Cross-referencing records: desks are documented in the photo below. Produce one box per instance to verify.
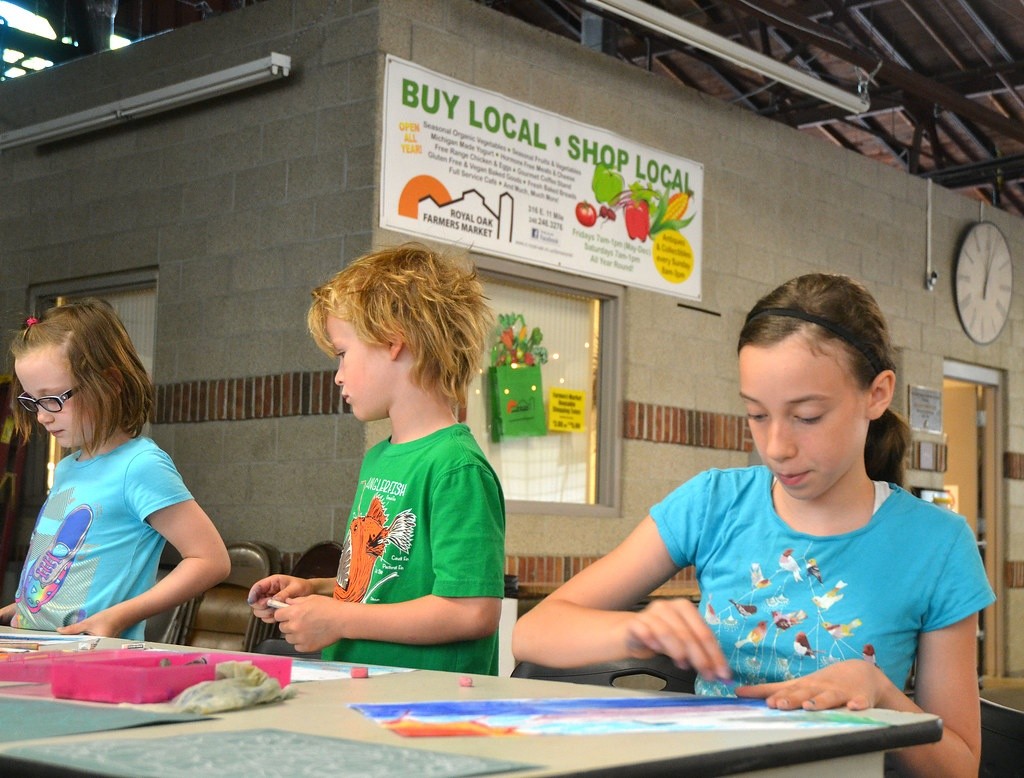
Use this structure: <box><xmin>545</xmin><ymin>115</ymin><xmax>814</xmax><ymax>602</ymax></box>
<box><xmin>0</xmin><ymin>626</ymin><xmax>943</xmax><ymax>778</ymax></box>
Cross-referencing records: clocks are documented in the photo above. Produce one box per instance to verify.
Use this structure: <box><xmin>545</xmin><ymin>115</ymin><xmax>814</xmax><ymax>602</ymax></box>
<box><xmin>955</xmin><ymin>222</ymin><xmax>1012</xmax><ymax>344</ymax></box>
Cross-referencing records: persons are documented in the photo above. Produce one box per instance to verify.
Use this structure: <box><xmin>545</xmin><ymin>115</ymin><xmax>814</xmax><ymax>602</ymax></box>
<box><xmin>512</xmin><ymin>273</ymin><xmax>996</xmax><ymax>778</ymax></box>
<box><xmin>247</xmin><ymin>242</ymin><xmax>508</xmax><ymax>678</ymax></box>
<box><xmin>0</xmin><ymin>297</ymin><xmax>231</xmax><ymax>637</ymax></box>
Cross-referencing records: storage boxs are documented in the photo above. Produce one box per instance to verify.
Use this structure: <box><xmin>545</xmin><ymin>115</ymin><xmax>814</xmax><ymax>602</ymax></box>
<box><xmin>0</xmin><ymin>648</ymin><xmax>293</xmax><ymax>706</ymax></box>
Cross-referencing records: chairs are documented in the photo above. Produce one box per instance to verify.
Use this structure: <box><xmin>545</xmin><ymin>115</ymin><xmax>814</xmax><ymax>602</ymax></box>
<box><xmin>289</xmin><ymin>540</ymin><xmax>345</xmax><ymax>579</ymax></box>
<box><xmin>881</xmin><ymin>696</ymin><xmax>1024</xmax><ymax>778</ymax></box>
<box><xmin>163</xmin><ymin>540</ymin><xmax>282</xmax><ymax>653</ymax></box>
<box><xmin>509</xmin><ymin>650</ymin><xmax>698</xmax><ymax>696</ymax></box>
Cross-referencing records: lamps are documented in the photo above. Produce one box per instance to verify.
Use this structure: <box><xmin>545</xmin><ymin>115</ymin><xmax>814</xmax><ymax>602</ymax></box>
<box><xmin>0</xmin><ymin>52</ymin><xmax>290</xmax><ymax>151</ymax></box>
<box><xmin>586</xmin><ymin>0</ymin><xmax>870</xmax><ymax>114</ymax></box>
